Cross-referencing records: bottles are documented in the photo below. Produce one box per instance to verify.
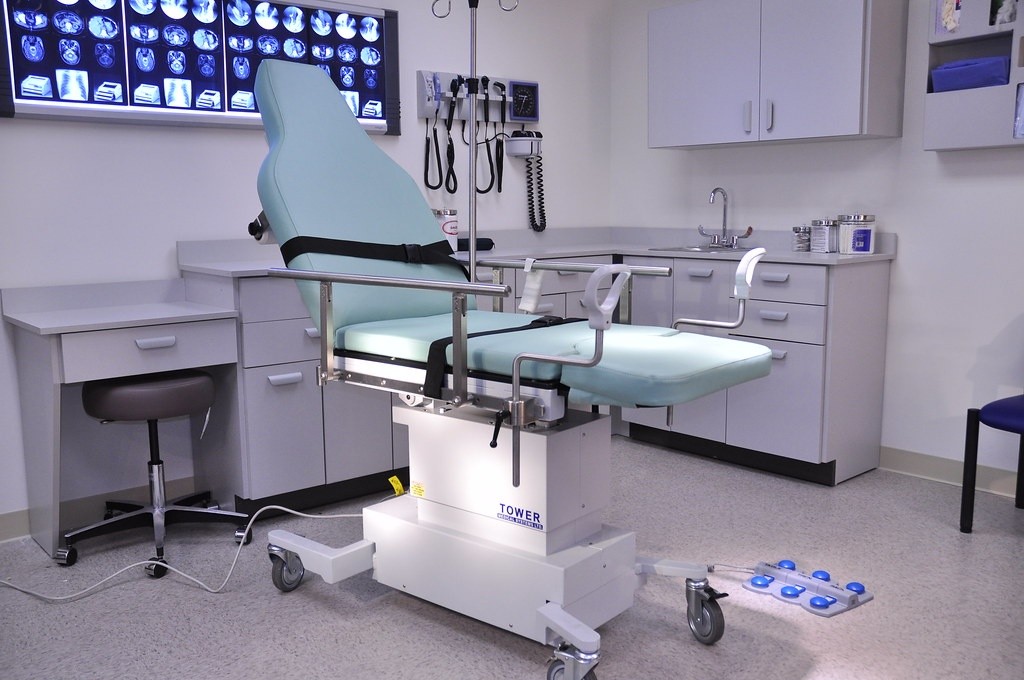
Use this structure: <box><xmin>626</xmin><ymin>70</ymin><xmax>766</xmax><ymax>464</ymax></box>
<box><xmin>443</xmin><ymin>210</ymin><xmax>458</xmax><ymax>252</ymax></box>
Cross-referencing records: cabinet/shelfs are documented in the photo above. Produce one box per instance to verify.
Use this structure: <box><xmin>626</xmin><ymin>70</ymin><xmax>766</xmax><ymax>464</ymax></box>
<box><xmin>392</xmin><ymin>267</ymin><xmax>518</xmax><ymax>487</ymax></box>
<box><xmin>620</xmin><ymin>256</ymin><xmax>731</xmax><ymax>461</ymax></box>
<box><xmin>237</xmin><ymin>276</ymin><xmax>394</xmax><ymax>521</ymax></box>
<box><xmin>725</xmin><ymin>260</ymin><xmax>891</xmax><ymax>487</ymax></box>
<box><xmin>921</xmin><ymin>0</ymin><xmax>1024</xmax><ymax>153</ymax></box>
<box><xmin>647</xmin><ymin>0</ymin><xmax>909</xmax><ymax>152</ymax></box>
<box><xmin>516</xmin><ymin>254</ymin><xmax>613</xmax><ymax>416</ymax></box>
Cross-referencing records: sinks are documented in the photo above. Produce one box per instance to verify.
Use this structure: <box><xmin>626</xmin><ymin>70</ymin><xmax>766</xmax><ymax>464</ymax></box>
<box><xmin>647</xmin><ymin>245</ymin><xmax>754</xmax><ymax>254</ymax></box>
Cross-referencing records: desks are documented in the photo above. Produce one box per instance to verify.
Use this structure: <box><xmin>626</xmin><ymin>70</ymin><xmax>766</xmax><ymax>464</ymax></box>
<box><xmin>0</xmin><ymin>276</ymin><xmax>239</xmax><ymax>558</ymax></box>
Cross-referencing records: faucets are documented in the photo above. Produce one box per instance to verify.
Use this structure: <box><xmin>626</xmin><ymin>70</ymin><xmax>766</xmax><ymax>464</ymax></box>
<box><xmin>709</xmin><ymin>187</ymin><xmax>728</xmax><ymax>244</ymax></box>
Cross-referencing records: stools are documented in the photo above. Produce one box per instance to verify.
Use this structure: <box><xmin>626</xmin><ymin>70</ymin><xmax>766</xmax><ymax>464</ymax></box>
<box><xmin>55</xmin><ymin>371</ymin><xmax>252</xmax><ymax>580</ymax></box>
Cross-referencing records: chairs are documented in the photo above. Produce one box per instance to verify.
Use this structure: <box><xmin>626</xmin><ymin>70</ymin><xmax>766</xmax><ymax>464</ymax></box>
<box><xmin>247</xmin><ymin>57</ymin><xmax>772</xmax><ymax>427</ymax></box>
<box><xmin>959</xmin><ymin>394</ymin><xmax>1024</xmax><ymax>535</ymax></box>
